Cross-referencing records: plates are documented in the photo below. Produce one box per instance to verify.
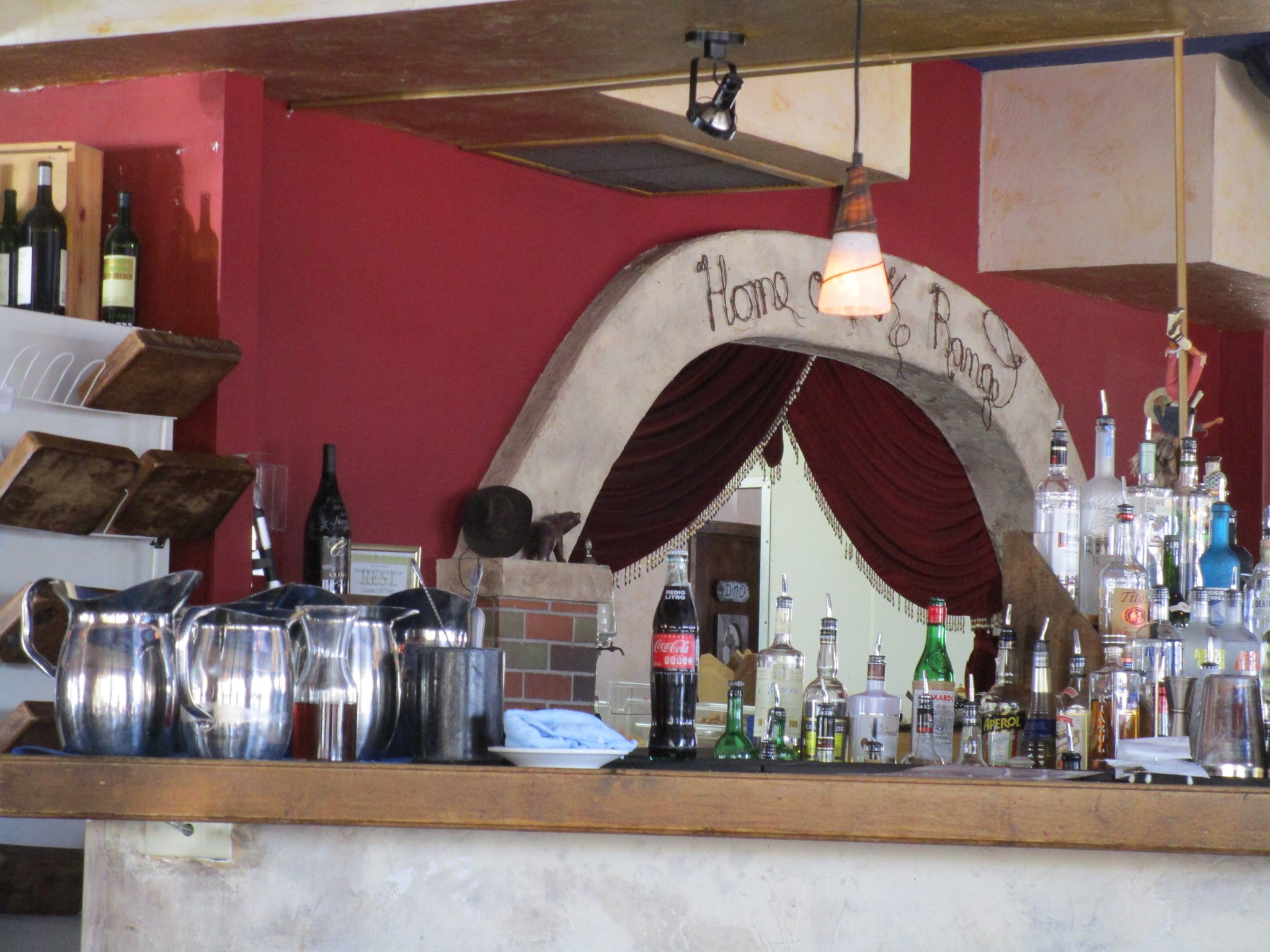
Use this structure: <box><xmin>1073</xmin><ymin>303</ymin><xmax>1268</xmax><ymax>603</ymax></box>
<box><xmin>487</xmin><ymin>746</ymin><xmax>630</xmax><ymax>772</ymax></box>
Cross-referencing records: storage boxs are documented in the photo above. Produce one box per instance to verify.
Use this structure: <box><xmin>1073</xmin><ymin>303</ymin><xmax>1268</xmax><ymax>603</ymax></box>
<box><xmin>0</xmin><ymin>143</ymin><xmax>102</xmax><ymax>321</ymax></box>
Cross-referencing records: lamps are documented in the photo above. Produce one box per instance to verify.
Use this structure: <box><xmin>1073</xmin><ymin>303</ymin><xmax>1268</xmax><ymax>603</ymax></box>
<box><xmin>817</xmin><ymin>1</ymin><xmax>891</xmax><ymax>316</ymax></box>
<box><xmin>681</xmin><ymin>29</ymin><xmax>749</xmax><ymax>142</ymax></box>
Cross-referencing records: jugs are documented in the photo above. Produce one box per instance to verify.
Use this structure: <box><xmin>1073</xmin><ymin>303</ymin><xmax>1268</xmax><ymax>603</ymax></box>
<box><xmin>18</xmin><ymin>569</ymin><xmax>415</xmax><ymax>761</ymax></box>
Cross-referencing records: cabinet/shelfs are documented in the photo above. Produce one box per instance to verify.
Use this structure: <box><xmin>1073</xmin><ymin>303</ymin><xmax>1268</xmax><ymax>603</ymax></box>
<box><xmin>0</xmin><ymin>303</ymin><xmax>173</xmax><ymax>916</ymax></box>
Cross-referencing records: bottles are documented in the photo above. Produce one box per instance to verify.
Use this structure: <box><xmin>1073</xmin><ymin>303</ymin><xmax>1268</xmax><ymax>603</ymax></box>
<box><xmin>301</xmin><ymin>443</ymin><xmax>350</xmax><ymax>594</ymax></box>
<box><xmin>252</xmin><ymin>501</ymin><xmax>280</xmax><ymax>593</ymax></box>
<box><xmin>956</xmin><ymin>388</ymin><xmax>1267</xmax><ymax>780</ymax></box>
<box><xmin>907</xmin><ymin>596</ymin><xmax>955</xmax><ymax>763</ymax></box>
<box><xmin>844</xmin><ymin>631</ymin><xmax>902</xmax><ymax>764</ymax></box>
<box><xmin>0</xmin><ymin>188</ymin><xmax>21</xmax><ymax>307</ymax></box>
<box><xmin>755</xmin><ymin>708</ymin><xmax>799</xmax><ymax>760</ymax></box>
<box><xmin>812</xmin><ymin>678</ymin><xmax>837</xmax><ymax>763</ymax></box>
<box><xmin>794</xmin><ymin>593</ymin><xmax>851</xmax><ymax>760</ymax></box>
<box><xmin>98</xmin><ymin>191</ymin><xmax>141</xmax><ymax>326</ymax></box>
<box><xmin>16</xmin><ymin>161</ymin><xmax>68</xmax><ymax>314</ymax></box>
<box><xmin>753</xmin><ymin>572</ymin><xmax>806</xmax><ymax>737</ymax></box>
<box><xmin>707</xmin><ymin>679</ymin><xmax>759</xmax><ymax>760</ymax></box>
<box><xmin>897</xmin><ymin>669</ymin><xmax>947</xmax><ymax>766</ymax></box>
<box><xmin>860</xmin><ymin>716</ymin><xmax>887</xmax><ymax>763</ymax></box>
<box><xmin>644</xmin><ymin>550</ymin><xmax>702</xmax><ymax>760</ymax></box>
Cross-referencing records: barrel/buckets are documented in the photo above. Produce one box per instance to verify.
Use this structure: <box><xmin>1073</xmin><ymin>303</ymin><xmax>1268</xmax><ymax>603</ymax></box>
<box><xmin>405</xmin><ymin>646</ymin><xmax>509</xmax><ymax>767</ymax></box>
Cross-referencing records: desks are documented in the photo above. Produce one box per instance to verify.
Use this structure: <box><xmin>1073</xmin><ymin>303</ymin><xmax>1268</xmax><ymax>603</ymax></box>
<box><xmin>0</xmin><ymin>748</ymin><xmax>1270</xmax><ymax>952</ymax></box>
